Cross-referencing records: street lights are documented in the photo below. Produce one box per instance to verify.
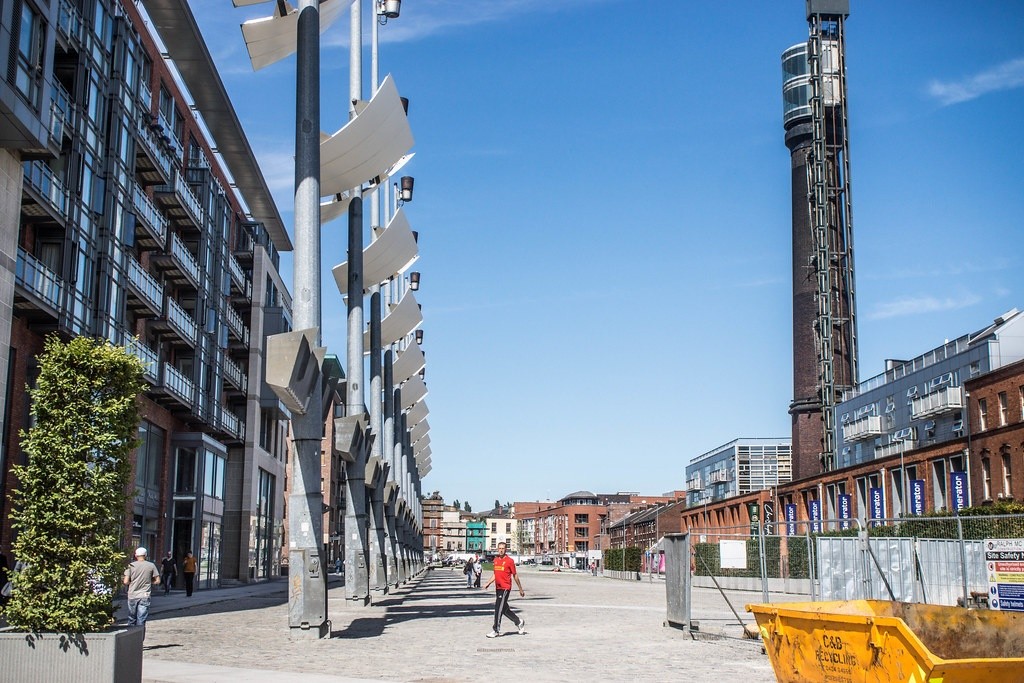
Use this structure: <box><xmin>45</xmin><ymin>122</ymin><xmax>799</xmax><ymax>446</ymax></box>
<box><xmin>232</xmin><ymin>1</ymin><xmax>434</xmax><ymax>632</ymax></box>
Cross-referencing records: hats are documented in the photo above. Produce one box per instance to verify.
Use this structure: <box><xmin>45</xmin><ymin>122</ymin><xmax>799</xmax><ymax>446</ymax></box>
<box><xmin>134</xmin><ymin>547</ymin><xmax>147</xmax><ymax>557</ymax></box>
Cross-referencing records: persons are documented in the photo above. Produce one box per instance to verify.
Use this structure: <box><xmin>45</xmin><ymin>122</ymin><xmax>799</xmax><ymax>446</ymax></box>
<box><xmin>484</xmin><ymin>542</ymin><xmax>525</xmax><ymax>638</ymax></box>
<box><xmin>0</xmin><ymin>545</ymin><xmax>28</xmax><ymax>606</ymax></box>
<box><xmin>123</xmin><ymin>547</ymin><xmax>161</xmax><ymax>641</ymax></box>
<box><xmin>161</xmin><ymin>551</ymin><xmax>178</xmax><ymax>597</ymax></box>
<box><xmin>466</xmin><ymin>558</ymin><xmax>482</xmax><ymax>589</ymax></box>
<box><xmin>183</xmin><ymin>550</ymin><xmax>197</xmax><ymax>596</ymax></box>
<box><xmin>336</xmin><ymin>558</ymin><xmax>342</xmax><ymax>574</ymax></box>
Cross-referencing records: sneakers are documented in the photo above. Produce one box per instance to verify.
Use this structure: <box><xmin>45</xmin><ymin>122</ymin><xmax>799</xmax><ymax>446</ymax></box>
<box><xmin>516</xmin><ymin>618</ymin><xmax>527</xmax><ymax>635</ymax></box>
<box><xmin>485</xmin><ymin>631</ymin><xmax>499</xmax><ymax>638</ymax></box>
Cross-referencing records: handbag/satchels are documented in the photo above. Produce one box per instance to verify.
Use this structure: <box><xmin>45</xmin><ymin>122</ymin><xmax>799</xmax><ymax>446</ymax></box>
<box><xmin>463</xmin><ymin>563</ymin><xmax>469</xmax><ymax>575</ymax></box>
